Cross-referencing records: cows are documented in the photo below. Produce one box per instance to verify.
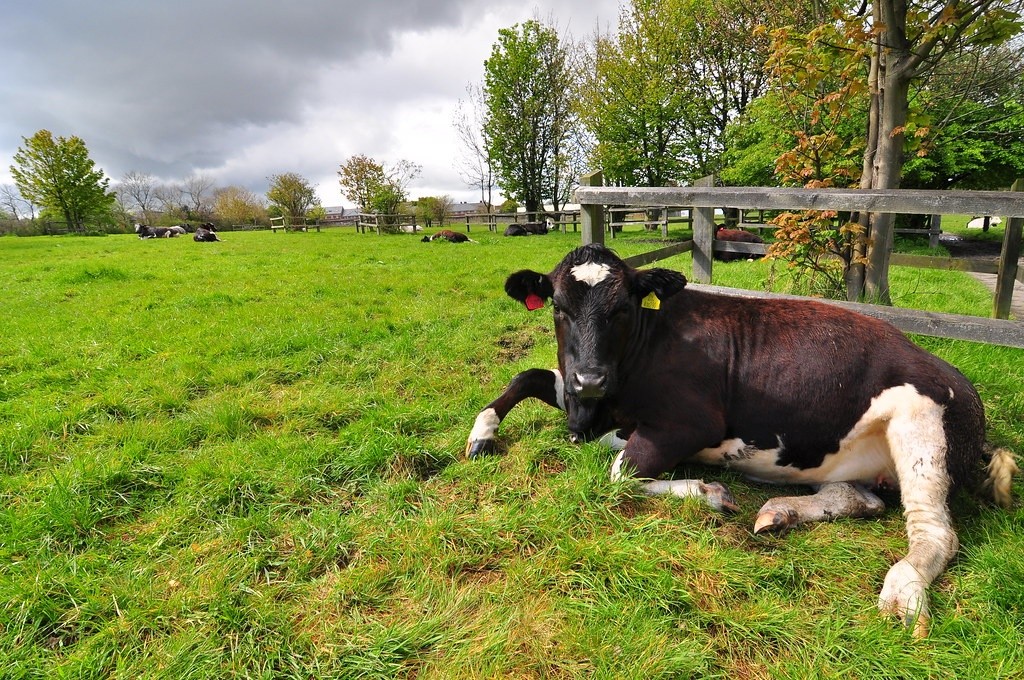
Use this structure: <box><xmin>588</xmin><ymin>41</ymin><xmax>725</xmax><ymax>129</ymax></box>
<box><xmin>502</xmin><ymin>217</ymin><xmax>556</xmax><ymax>237</ymax></box>
<box><xmin>397</xmin><ymin>223</ymin><xmax>423</xmax><ymax>233</ymax></box>
<box><xmin>462</xmin><ymin>240</ymin><xmax>1019</xmax><ymax>642</ymax></box>
<box><xmin>421</xmin><ymin>229</ymin><xmax>479</xmax><ymax>244</ymax></box>
<box><xmin>193</xmin><ymin>222</ymin><xmax>228</xmax><ymax>242</ymax></box>
<box><xmin>134</xmin><ymin>223</ymin><xmax>197</xmax><ymax>240</ymax></box>
